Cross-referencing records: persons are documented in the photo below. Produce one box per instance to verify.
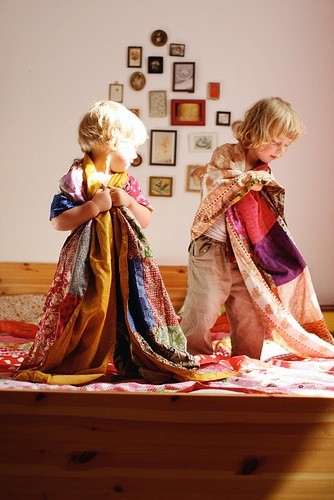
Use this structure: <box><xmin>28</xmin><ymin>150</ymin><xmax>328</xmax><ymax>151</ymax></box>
<box><xmin>49</xmin><ymin>100</ymin><xmax>187</xmax><ymax>383</ymax></box>
<box><xmin>179</xmin><ymin>99</ymin><xmax>301</xmax><ymax>360</ymax></box>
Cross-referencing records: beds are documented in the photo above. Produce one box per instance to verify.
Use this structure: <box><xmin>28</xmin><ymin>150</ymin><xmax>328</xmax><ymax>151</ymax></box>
<box><xmin>0</xmin><ymin>263</ymin><xmax>334</xmax><ymax>500</ymax></box>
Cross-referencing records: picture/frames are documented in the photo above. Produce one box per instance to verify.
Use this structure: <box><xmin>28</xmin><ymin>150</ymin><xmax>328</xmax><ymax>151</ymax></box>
<box><xmin>108</xmin><ymin>30</ymin><xmax>231</xmax><ymax>197</ymax></box>
